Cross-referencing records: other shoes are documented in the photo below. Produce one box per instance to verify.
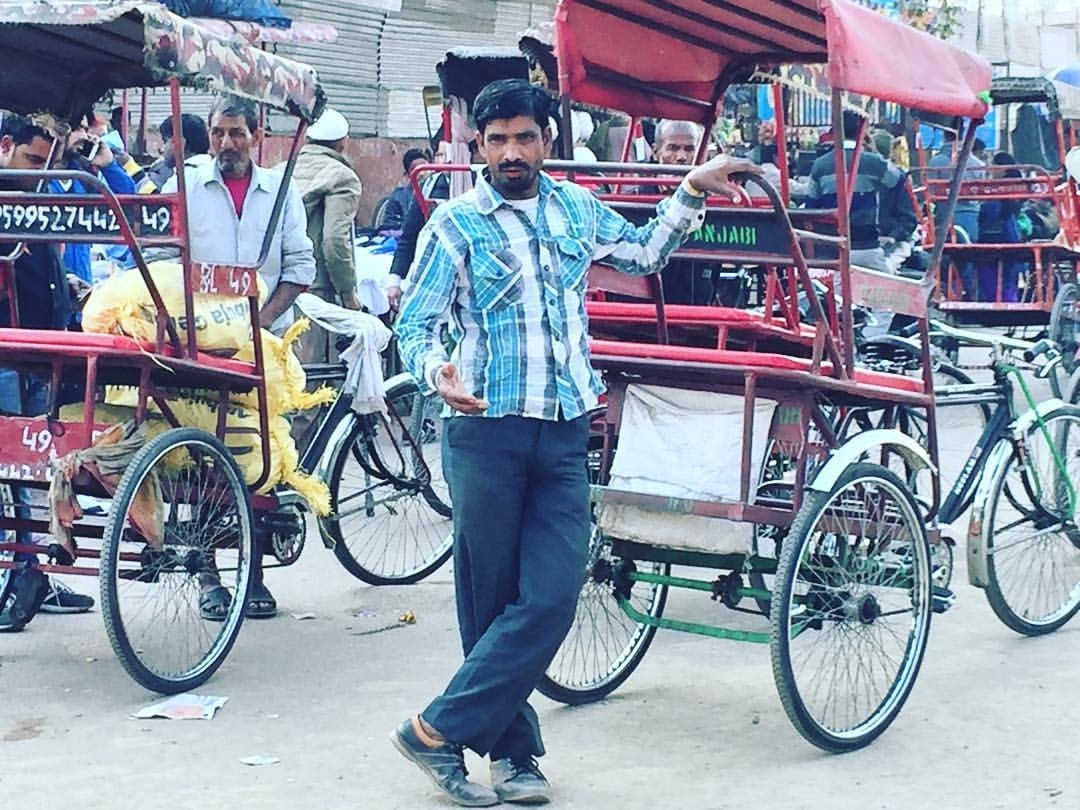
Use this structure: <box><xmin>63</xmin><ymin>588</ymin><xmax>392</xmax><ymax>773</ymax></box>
<box><xmin>388</xmin><ymin>712</ymin><xmax>500</xmax><ymax>806</ymax></box>
<box><xmin>490</xmin><ymin>756</ymin><xmax>551</xmax><ymax>805</ymax></box>
<box><xmin>401</xmin><ymin>421</ymin><xmax>437</xmax><ymax>447</ymax></box>
<box><xmin>38</xmin><ymin>576</ymin><xmax>95</xmax><ymax>613</ymax></box>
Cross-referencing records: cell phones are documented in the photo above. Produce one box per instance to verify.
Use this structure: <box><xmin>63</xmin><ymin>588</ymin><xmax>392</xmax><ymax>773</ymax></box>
<box><xmin>73</xmin><ymin>138</ymin><xmax>99</xmax><ymax>160</ymax></box>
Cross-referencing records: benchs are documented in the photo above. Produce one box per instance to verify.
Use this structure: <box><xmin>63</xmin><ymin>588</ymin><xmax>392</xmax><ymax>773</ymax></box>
<box><xmin>931</xmin><ymin>176</ymin><xmax>1080</xmax><ymax>305</ymax></box>
<box><xmin>413</xmin><ymin>164</ymin><xmax>854</xmax><ymax>378</ymax></box>
<box><xmin>0</xmin><ymin>191</ymin><xmax>272</xmax><ymax>491</ymax></box>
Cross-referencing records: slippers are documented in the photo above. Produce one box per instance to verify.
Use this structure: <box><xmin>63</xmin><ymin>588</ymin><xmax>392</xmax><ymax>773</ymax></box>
<box><xmin>244</xmin><ymin>584</ymin><xmax>277</xmax><ymax>618</ymax></box>
<box><xmin>197</xmin><ymin>586</ymin><xmax>234</xmax><ymax>621</ymax></box>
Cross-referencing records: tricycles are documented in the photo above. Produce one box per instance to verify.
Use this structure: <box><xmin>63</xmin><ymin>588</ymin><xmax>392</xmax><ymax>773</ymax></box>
<box><xmin>0</xmin><ymin>0</ymin><xmax>454</xmax><ymax>694</ymax></box>
<box><xmin>901</xmin><ymin>75</ymin><xmax>1080</xmax><ymax>405</ymax></box>
<box><xmin>406</xmin><ymin>0</ymin><xmax>1078</xmax><ymax>754</ymax></box>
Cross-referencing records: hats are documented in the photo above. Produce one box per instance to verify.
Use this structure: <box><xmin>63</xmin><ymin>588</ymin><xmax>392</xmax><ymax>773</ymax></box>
<box><xmin>306</xmin><ymin>108</ymin><xmax>350</xmax><ymax>141</ymax></box>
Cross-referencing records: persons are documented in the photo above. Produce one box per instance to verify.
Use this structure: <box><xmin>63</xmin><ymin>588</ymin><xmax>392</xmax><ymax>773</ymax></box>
<box><xmin>271</xmin><ymin>108</ymin><xmax>380</xmax><ymax>464</ymax></box>
<box><xmin>612</xmin><ymin>104</ymin><xmax>1026</xmax><ymax>337</ymax></box>
<box><xmin>161</xmin><ymin>95</ymin><xmax>318</xmax><ymax>619</ymax></box>
<box><xmin>387</xmin><ymin>79</ymin><xmax>764</xmax><ymax>806</ymax></box>
<box><xmin>0</xmin><ymin>100</ymin><xmax>212</xmax><ymax>616</ymax></box>
<box><xmin>382</xmin><ymin>130</ymin><xmax>474</xmax><ymax>447</ymax></box>
